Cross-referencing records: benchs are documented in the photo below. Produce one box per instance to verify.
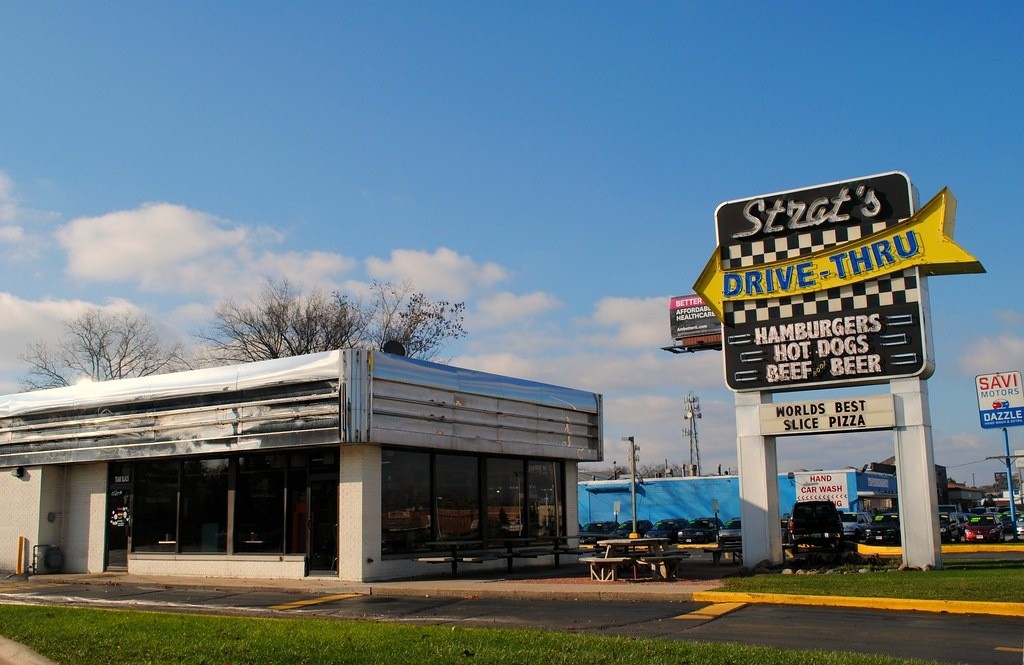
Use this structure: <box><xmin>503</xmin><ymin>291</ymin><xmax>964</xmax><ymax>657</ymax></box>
<box><xmin>416</xmin><ymin>541</ymin><xmax>692</xmax><ymax>581</ymax></box>
<box><xmin>704</xmin><ymin>535</ymin><xmax>743</xmax><ymax>566</ymax></box>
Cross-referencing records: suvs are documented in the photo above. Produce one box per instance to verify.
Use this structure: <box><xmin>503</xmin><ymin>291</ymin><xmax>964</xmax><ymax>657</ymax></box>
<box><xmin>787</xmin><ymin>500</ymin><xmax>844</xmax><ymax>551</ymax></box>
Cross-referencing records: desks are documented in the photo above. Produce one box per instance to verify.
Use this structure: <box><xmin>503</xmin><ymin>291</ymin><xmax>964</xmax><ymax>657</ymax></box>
<box><xmin>596</xmin><ymin>537</ymin><xmax>672</xmax><ymax>582</ymax></box>
<box><xmin>577</xmin><ymin>532</ymin><xmax>620</xmax><ymax>554</ymax></box>
<box><xmin>424</xmin><ymin>540</ymin><xmax>480</xmax><ymax>577</ymax></box>
<box><xmin>536</xmin><ymin>534</ymin><xmax>581</xmax><ymax>569</ymax></box>
<box><xmin>484</xmin><ymin>537</ymin><xmax>537</xmax><ymax>572</ymax></box>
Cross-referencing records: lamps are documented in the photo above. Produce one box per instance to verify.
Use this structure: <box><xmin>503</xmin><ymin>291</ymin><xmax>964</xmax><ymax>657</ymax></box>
<box><xmin>11</xmin><ymin>466</ymin><xmax>25</xmax><ymax>477</ymax></box>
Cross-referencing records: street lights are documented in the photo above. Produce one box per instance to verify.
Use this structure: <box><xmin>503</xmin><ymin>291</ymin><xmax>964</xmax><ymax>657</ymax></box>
<box><xmin>620</xmin><ymin>436</ymin><xmax>642</xmax><ymax>539</ymax></box>
<box><xmin>435</xmin><ymin>496</ymin><xmax>443</xmax><ymax>538</ymax></box>
<box><xmin>514</xmin><ymin>470</ymin><xmax>523</xmax><ymax>538</ymax></box>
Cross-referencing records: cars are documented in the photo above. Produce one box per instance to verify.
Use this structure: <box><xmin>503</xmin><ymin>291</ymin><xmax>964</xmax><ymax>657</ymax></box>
<box><xmin>531</xmin><ymin>524</ymin><xmax>551</xmax><ymax>537</ymax></box>
<box><xmin>714</xmin><ymin>519</ymin><xmax>743</xmax><ymax>544</ymax></box>
<box><xmin>677</xmin><ymin>517</ymin><xmax>728</xmax><ymax>545</ymax></box>
<box><xmin>842</xmin><ymin>504</ymin><xmax>1024</xmax><ymax>545</ymax></box>
<box><xmin>644</xmin><ymin>517</ymin><xmax>689</xmax><ymax>543</ymax></box>
<box><xmin>608</xmin><ymin>520</ymin><xmax>654</xmax><ymax>540</ymax></box>
<box><xmin>578</xmin><ymin>521</ymin><xmax>621</xmax><ymax>544</ymax></box>
<box><xmin>501</xmin><ymin>519</ymin><xmax>524</xmax><ymax>534</ymax></box>
